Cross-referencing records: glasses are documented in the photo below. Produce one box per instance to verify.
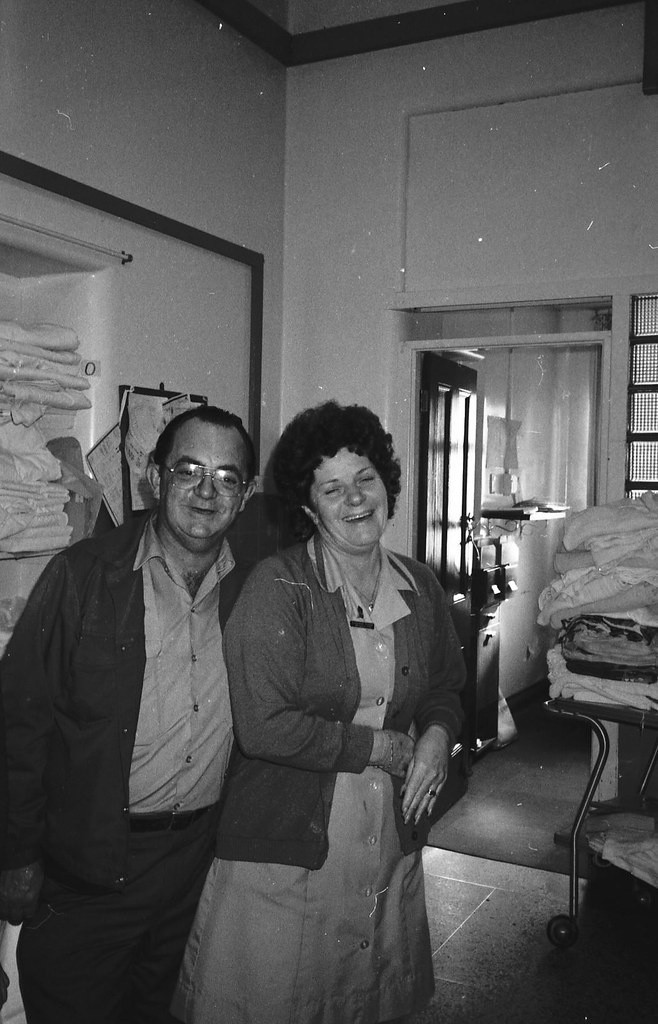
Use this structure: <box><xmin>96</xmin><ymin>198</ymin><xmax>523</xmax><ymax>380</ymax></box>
<box><xmin>159</xmin><ymin>455</ymin><xmax>253</xmax><ymax>498</ymax></box>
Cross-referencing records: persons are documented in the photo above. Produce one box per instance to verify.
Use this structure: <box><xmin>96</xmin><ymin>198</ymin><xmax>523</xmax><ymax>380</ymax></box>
<box><xmin>0</xmin><ymin>408</ymin><xmax>314</xmax><ymax>1024</ymax></box>
<box><xmin>168</xmin><ymin>400</ymin><xmax>469</xmax><ymax>1024</ymax></box>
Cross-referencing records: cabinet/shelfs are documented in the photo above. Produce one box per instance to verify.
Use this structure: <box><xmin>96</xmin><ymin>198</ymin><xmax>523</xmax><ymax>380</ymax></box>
<box><xmin>482</xmin><ymin>505</ymin><xmax>566</xmax><ymax>541</ymax></box>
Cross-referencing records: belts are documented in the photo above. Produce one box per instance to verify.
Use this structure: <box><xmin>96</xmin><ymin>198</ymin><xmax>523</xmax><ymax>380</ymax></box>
<box><xmin>130</xmin><ymin>806</ymin><xmax>207</xmax><ymax>833</ymax></box>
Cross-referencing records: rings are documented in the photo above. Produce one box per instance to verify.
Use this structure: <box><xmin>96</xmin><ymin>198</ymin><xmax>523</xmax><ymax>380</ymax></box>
<box><xmin>427</xmin><ymin>789</ymin><xmax>436</xmax><ymax>797</ymax></box>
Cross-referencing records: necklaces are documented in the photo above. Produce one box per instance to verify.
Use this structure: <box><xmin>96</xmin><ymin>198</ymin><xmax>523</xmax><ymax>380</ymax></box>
<box><xmin>347</xmin><ymin>566</ymin><xmax>381</xmax><ymax>612</ymax></box>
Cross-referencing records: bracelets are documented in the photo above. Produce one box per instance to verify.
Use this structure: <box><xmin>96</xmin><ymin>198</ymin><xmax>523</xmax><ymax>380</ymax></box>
<box><xmin>373</xmin><ymin>730</ymin><xmax>393</xmax><ymax>769</ymax></box>
